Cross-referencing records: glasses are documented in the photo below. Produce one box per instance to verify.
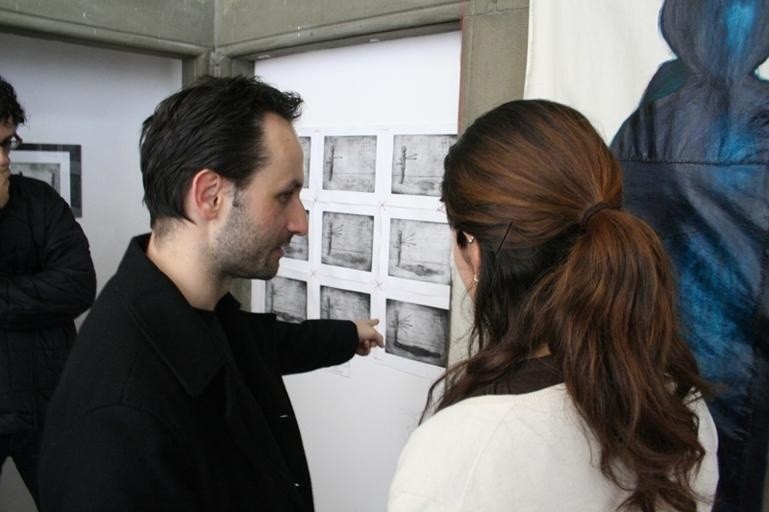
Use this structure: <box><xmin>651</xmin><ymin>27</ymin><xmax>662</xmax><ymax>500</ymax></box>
<box><xmin>1</xmin><ymin>133</ymin><xmax>23</xmax><ymax>150</ymax></box>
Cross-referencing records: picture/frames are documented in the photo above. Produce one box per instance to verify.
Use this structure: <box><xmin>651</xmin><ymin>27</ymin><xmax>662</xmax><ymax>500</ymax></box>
<box><xmin>7</xmin><ymin>143</ymin><xmax>82</xmax><ymax>218</ymax></box>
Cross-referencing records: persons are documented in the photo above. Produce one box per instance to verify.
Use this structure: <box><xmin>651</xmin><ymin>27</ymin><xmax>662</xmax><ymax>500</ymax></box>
<box><xmin>385</xmin><ymin>99</ymin><xmax>720</xmax><ymax>511</ymax></box>
<box><xmin>611</xmin><ymin>0</ymin><xmax>768</xmax><ymax>512</ymax></box>
<box><xmin>35</xmin><ymin>70</ymin><xmax>385</xmax><ymax>511</ymax></box>
<box><xmin>0</xmin><ymin>73</ymin><xmax>97</xmax><ymax>512</ymax></box>
